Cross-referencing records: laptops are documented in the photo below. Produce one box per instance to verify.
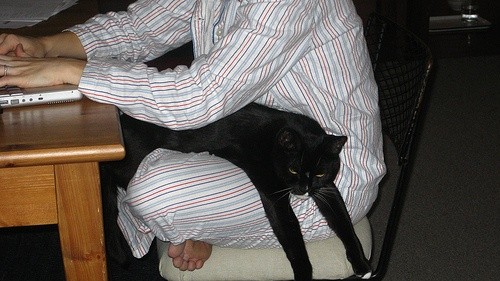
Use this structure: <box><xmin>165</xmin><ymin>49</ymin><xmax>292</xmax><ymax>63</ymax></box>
<box><xmin>0</xmin><ymin>83</ymin><xmax>83</xmax><ymax>109</ymax></box>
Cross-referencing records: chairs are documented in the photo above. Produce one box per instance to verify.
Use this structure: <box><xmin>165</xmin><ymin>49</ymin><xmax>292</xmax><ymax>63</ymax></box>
<box><xmin>156</xmin><ymin>11</ymin><xmax>434</xmax><ymax>281</ymax></box>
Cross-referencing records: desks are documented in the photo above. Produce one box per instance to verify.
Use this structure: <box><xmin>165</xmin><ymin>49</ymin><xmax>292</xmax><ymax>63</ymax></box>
<box><xmin>0</xmin><ymin>0</ymin><xmax>126</xmax><ymax>281</ymax></box>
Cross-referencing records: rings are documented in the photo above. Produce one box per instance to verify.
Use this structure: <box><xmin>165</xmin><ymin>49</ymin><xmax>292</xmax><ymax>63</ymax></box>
<box><xmin>4</xmin><ymin>65</ymin><xmax>7</xmax><ymax>76</ymax></box>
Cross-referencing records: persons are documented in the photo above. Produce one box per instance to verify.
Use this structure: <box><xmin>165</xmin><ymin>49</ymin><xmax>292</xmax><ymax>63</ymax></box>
<box><xmin>0</xmin><ymin>0</ymin><xmax>386</xmax><ymax>272</ymax></box>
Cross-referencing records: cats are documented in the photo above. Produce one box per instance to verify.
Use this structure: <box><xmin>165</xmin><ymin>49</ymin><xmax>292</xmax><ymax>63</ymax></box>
<box><xmin>99</xmin><ymin>101</ymin><xmax>373</xmax><ymax>281</ymax></box>
